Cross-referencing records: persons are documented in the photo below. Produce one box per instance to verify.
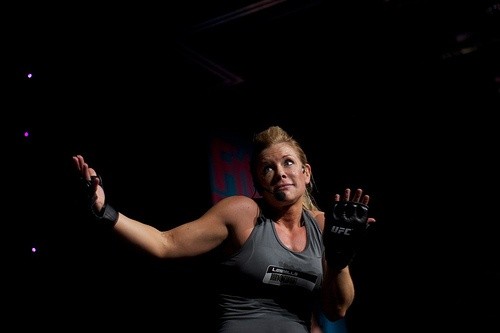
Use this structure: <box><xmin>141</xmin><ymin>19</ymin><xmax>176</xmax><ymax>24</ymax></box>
<box><xmin>73</xmin><ymin>124</ymin><xmax>376</xmax><ymax>332</ymax></box>
